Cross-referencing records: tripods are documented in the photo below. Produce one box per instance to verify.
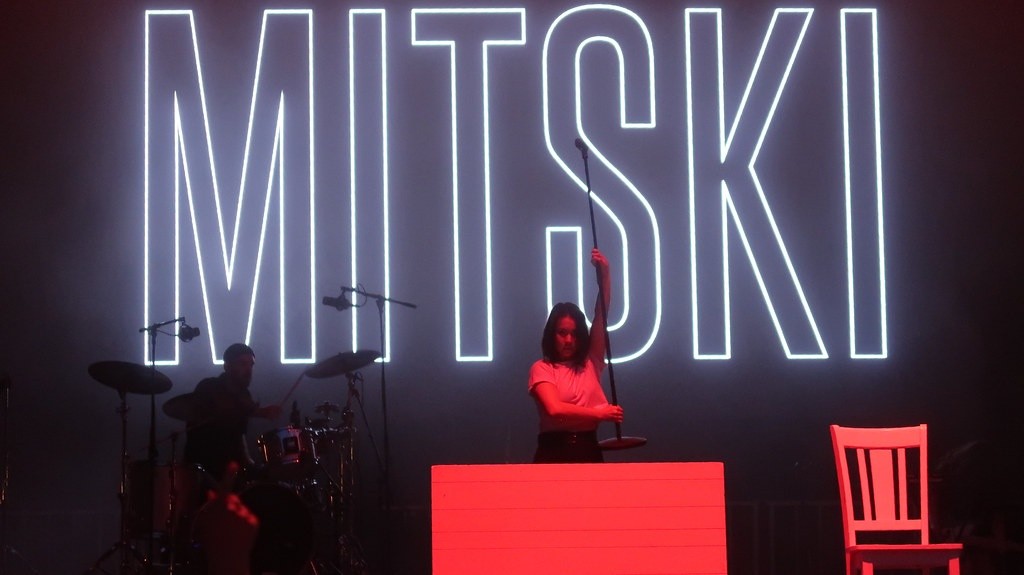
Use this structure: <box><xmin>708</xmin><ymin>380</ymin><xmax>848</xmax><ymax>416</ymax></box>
<box><xmin>299</xmin><ymin>422</ymin><xmax>376</xmax><ymax>575</ymax></box>
<box><xmin>91</xmin><ymin>383</ymin><xmax>153</xmax><ymax>575</ymax></box>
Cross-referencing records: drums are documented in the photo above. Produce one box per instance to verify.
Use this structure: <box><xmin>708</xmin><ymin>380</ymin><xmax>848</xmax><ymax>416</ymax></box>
<box><xmin>259</xmin><ymin>426</ymin><xmax>312</xmax><ymax>471</ymax></box>
<box><xmin>124</xmin><ymin>457</ymin><xmax>187</xmax><ymax>547</ymax></box>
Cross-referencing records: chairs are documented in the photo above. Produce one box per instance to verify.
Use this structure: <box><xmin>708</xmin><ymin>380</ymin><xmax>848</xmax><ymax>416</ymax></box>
<box><xmin>831</xmin><ymin>424</ymin><xmax>964</xmax><ymax>575</ymax></box>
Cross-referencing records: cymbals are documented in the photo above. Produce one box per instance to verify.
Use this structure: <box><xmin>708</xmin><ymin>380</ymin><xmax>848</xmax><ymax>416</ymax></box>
<box><xmin>162</xmin><ymin>391</ymin><xmax>193</xmax><ymax>420</ymax></box>
<box><xmin>304</xmin><ymin>350</ymin><xmax>382</xmax><ymax>378</ymax></box>
<box><xmin>87</xmin><ymin>359</ymin><xmax>173</xmax><ymax>396</ymax></box>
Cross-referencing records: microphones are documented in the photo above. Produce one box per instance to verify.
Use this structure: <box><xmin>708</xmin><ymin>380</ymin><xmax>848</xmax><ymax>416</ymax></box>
<box><xmin>177</xmin><ymin>325</ymin><xmax>200</xmax><ymax>341</ymax></box>
<box><xmin>321</xmin><ymin>295</ymin><xmax>353</xmax><ymax>310</ymax></box>
<box><xmin>574</xmin><ymin>137</ymin><xmax>589</xmax><ymax>153</ymax></box>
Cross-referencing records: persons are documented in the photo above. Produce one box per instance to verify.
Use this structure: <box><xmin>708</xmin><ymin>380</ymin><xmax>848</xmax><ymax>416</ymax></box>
<box><xmin>528</xmin><ymin>248</ymin><xmax>622</xmax><ymax>463</ymax></box>
<box><xmin>182</xmin><ymin>344</ymin><xmax>281</xmax><ymax>504</ymax></box>
